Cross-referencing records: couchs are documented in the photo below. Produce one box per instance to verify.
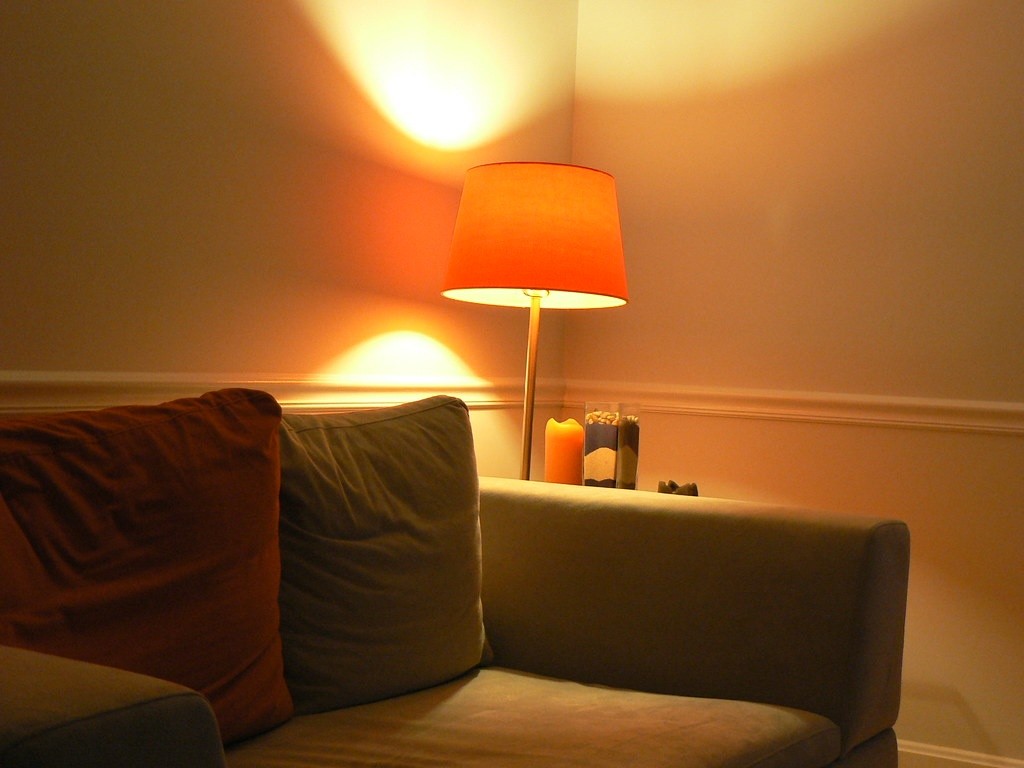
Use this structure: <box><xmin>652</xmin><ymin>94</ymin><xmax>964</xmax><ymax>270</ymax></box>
<box><xmin>0</xmin><ymin>474</ymin><xmax>916</xmax><ymax>768</ymax></box>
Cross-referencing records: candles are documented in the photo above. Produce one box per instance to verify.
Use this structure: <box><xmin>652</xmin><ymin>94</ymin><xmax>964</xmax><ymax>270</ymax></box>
<box><xmin>544</xmin><ymin>416</ymin><xmax>584</xmax><ymax>486</ymax></box>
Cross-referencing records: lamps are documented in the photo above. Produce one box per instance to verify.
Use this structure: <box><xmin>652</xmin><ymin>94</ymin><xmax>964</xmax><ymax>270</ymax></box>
<box><xmin>442</xmin><ymin>161</ymin><xmax>631</xmax><ymax>481</ymax></box>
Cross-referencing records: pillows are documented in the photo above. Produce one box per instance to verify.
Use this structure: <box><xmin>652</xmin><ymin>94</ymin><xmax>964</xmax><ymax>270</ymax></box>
<box><xmin>280</xmin><ymin>389</ymin><xmax>496</xmax><ymax>724</ymax></box>
<box><xmin>0</xmin><ymin>388</ymin><xmax>294</xmax><ymax>752</ymax></box>
